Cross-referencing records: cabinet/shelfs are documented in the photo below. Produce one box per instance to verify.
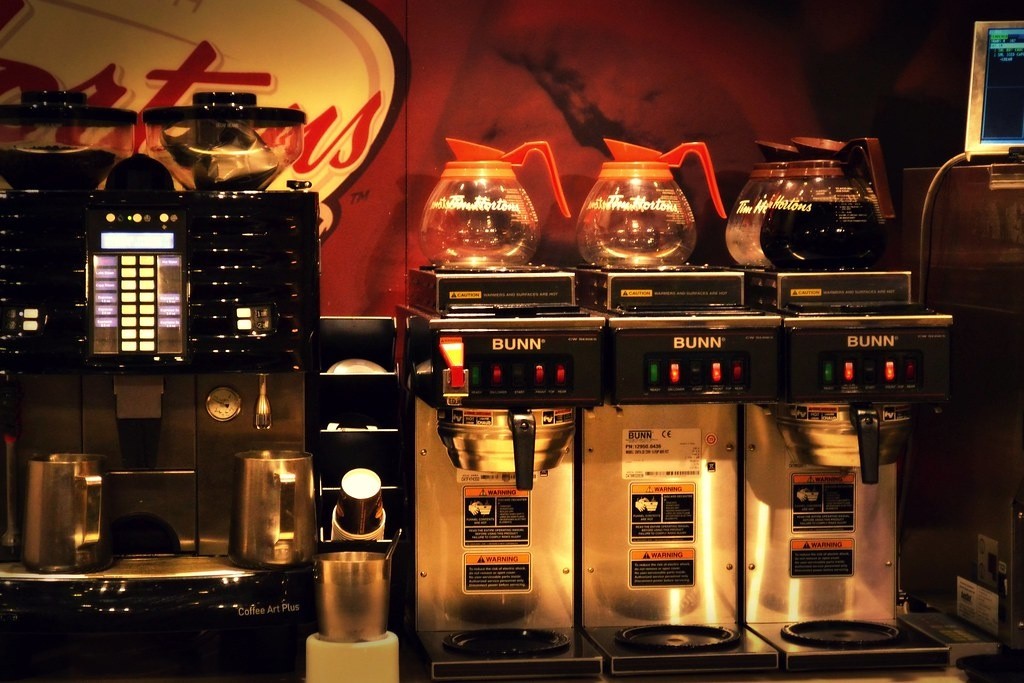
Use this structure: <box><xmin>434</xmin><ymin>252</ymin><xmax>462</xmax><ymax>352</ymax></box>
<box><xmin>314</xmin><ymin>316</ymin><xmax>399</xmax><ymax>545</ymax></box>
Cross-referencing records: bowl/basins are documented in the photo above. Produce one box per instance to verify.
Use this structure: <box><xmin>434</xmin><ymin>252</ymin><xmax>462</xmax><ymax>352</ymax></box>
<box><xmin>141</xmin><ymin>103</ymin><xmax>307</xmax><ymax>193</ymax></box>
<box><xmin>0</xmin><ymin>89</ymin><xmax>139</xmax><ymax>193</ymax></box>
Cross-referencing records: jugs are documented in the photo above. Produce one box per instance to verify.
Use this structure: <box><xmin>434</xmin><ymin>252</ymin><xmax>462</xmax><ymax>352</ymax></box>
<box><xmin>415</xmin><ymin>137</ymin><xmax>570</xmax><ymax>268</ymax></box>
<box><xmin>724</xmin><ymin>135</ymin><xmax>897</xmax><ymax>271</ymax></box>
<box><xmin>574</xmin><ymin>136</ymin><xmax>728</xmax><ymax>270</ymax></box>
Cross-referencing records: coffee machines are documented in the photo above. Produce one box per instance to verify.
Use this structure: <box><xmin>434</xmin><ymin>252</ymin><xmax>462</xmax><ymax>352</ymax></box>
<box><xmin>0</xmin><ymin>188</ymin><xmax>320</xmax><ymax>631</ymax></box>
<box><xmin>578</xmin><ymin>309</ymin><xmax>781</xmax><ymax>675</ymax></box>
<box><xmin>397</xmin><ymin>305</ymin><xmax>605</xmax><ymax>681</ymax></box>
<box><xmin>743</xmin><ymin>313</ymin><xmax>954</xmax><ymax>672</ymax></box>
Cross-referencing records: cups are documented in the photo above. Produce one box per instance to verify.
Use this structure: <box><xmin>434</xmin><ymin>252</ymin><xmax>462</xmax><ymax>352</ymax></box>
<box><xmin>23</xmin><ymin>453</ymin><xmax>114</xmax><ymax>572</ymax></box>
<box><xmin>314</xmin><ymin>551</ymin><xmax>389</xmax><ymax>640</ymax></box>
<box><xmin>228</xmin><ymin>449</ymin><xmax>318</xmax><ymax>569</ymax></box>
<box><xmin>329</xmin><ymin>467</ymin><xmax>387</xmax><ymax>541</ymax></box>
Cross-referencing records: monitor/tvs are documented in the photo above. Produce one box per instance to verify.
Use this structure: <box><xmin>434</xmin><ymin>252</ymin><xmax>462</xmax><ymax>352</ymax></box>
<box><xmin>963</xmin><ymin>21</ymin><xmax>1024</xmax><ymax>161</ymax></box>
